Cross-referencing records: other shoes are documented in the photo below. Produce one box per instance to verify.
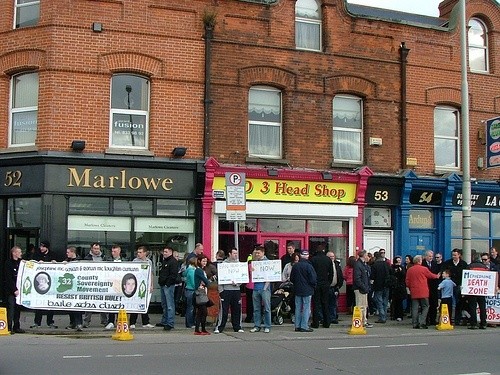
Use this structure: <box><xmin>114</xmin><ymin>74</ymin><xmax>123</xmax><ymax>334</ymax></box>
<box><xmin>12</xmin><ymin>325</ymin><xmax>25</xmax><ymax>333</ymax></box>
<box><xmin>164</xmin><ymin>324</ymin><xmax>174</xmax><ymax>330</ymax></box>
<box><xmin>201</xmin><ymin>330</ymin><xmax>211</xmax><ymax>335</ymax></box>
<box><xmin>156</xmin><ymin>322</ymin><xmax>165</xmax><ymax>327</ymax></box>
<box><xmin>105</xmin><ymin>322</ymin><xmax>115</xmax><ymax>330</ymax></box>
<box><xmin>29</xmin><ymin>324</ymin><xmax>42</xmax><ymax>329</ymax></box>
<box><xmin>142</xmin><ymin>323</ymin><xmax>154</xmax><ymax>328</ymax></box>
<box><xmin>100</xmin><ymin>321</ymin><xmax>109</xmax><ymax>326</ymax></box>
<box><xmin>47</xmin><ymin>323</ymin><xmax>59</xmax><ymax>329</ymax></box>
<box><xmin>130</xmin><ymin>324</ymin><xmax>136</xmax><ymax>329</ymax></box>
<box><xmin>8</xmin><ymin>329</ymin><xmax>14</xmax><ymax>335</ymax></box>
<box><xmin>76</xmin><ymin>323</ymin><xmax>83</xmax><ymax>331</ymax></box>
<box><xmin>194</xmin><ymin>330</ymin><xmax>201</xmax><ymax>335</ymax></box>
<box><xmin>83</xmin><ymin>319</ymin><xmax>90</xmax><ymax>328</ymax></box>
<box><xmin>212</xmin><ymin>312</ymin><xmax>489</xmax><ymax>334</ymax></box>
<box><xmin>185</xmin><ymin>324</ymin><xmax>193</xmax><ymax>328</ymax></box>
<box><xmin>65</xmin><ymin>323</ymin><xmax>76</xmax><ymax>330</ymax></box>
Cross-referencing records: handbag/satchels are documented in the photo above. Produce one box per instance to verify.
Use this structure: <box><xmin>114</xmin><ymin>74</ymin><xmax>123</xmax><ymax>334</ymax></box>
<box><xmin>196</xmin><ymin>289</ymin><xmax>209</xmax><ymax>304</ymax></box>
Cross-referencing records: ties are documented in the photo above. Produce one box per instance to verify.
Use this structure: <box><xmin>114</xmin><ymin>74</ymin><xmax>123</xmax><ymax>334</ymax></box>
<box><xmin>429</xmin><ymin>261</ymin><xmax>430</xmax><ymax>267</ymax></box>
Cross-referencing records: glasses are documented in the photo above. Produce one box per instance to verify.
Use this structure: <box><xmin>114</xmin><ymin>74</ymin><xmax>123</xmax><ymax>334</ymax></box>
<box><xmin>482</xmin><ymin>258</ymin><xmax>487</xmax><ymax>261</ymax></box>
<box><xmin>381</xmin><ymin>252</ymin><xmax>385</xmax><ymax>254</ymax></box>
<box><xmin>434</xmin><ymin>257</ymin><xmax>439</xmax><ymax>258</ymax></box>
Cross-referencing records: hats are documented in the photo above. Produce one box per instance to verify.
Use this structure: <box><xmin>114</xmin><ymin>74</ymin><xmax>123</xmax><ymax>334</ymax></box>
<box><xmin>40</xmin><ymin>240</ymin><xmax>50</xmax><ymax>248</ymax></box>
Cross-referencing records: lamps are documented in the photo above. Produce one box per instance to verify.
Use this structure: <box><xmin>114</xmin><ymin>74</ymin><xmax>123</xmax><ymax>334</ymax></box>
<box><xmin>268</xmin><ymin>167</ymin><xmax>278</xmax><ymax>176</ymax></box>
<box><xmin>320</xmin><ymin>169</ymin><xmax>333</xmax><ymax>180</ymax></box>
<box><xmin>173</xmin><ymin>148</ymin><xmax>187</xmax><ymax>156</ymax></box>
<box><xmin>71</xmin><ymin>140</ymin><xmax>86</xmax><ymax>150</ymax></box>
<box><xmin>93</xmin><ymin>22</ymin><xmax>103</xmax><ymax>32</ymax></box>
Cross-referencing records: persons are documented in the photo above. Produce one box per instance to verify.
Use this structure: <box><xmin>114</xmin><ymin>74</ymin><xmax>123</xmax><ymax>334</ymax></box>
<box><xmin>83</xmin><ymin>243</ymin><xmax>107</xmax><ymax>328</ymax></box>
<box><xmin>343</xmin><ymin>249</ymin><xmax>468</xmax><ymax>325</ymax></box>
<box><xmin>280</xmin><ymin>242</ymin><xmax>344</xmax><ymax>331</ymax></box>
<box><xmin>181</xmin><ymin>243</ymin><xmax>225</xmax><ymax>335</ymax></box>
<box><xmin>63</xmin><ymin>246</ymin><xmax>83</xmax><ymax>330</ymax></box>
<box><xmin>4</xmin><ymin>246</ymin><xmax>25</xmax><ymax>333</ymax></box>
<box><xmin>130</xmin><ymin>246</ymin><xmax>155</xmax><ymax>328</ymax></box>
<box><xmin>156</xmin><ymin>248</ymin><xmax>178</xmax><ymax>330</ymax></box>
<box><xmin>353</xmin><ymin>250</ymin><xmax>373</xmax><ymax>328</ymax></box>
<box><xmin>123</xmin><ymin>274</ymin><xmax>135</xmax><ymax>297</ymax></box>
<box><xmin>468</xmin><ymin>246</ymin><xmax>500</xmax><ymax>329</ymax></box>
<box><xmin>30</xmin><ymin>241</ymin><xmax>58</xmax><ymax>328</ymax></box>
<box><xmin>245</xmin><ymin>242</ymin><xmax>272</xmax><ymax>332</ymax></box>
<box><xmin>214</xmin><ymin>247</ymin><xmax>244</xmax><ymax>333</ymax></box>
<box><xmin>105</xmin><ymin>245</ymin><xmax>128</xmax><ymax>329</ymax></box>
<box><xmin>406</xmin><ymin>254</ymin><xmax>440</xmax><ymax>329</ymax></box>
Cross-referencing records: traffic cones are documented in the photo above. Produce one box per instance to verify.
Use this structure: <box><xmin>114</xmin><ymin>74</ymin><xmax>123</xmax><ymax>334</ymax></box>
<box><xmin>0</xmin><ymin>308</ymin><xmax>11</xmax><ymax>336</ymax></box>
<box><xmin>347</xmin><ymin>307</ymin><xmax>366</xmax><ymax>334</ymax></box>
<box><xmin>112</xmin><ymin>309</ymin><xmax>134</xmax><ymax>340</ymax></box>
<box><xmin>436</xmin><ymin>304</ymin><xmax>454</xmax><ymax>330</ymax></box>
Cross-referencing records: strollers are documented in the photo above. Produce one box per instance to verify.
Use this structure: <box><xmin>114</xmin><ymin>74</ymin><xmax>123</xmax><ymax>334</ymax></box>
<box><xmin>262</xmin><ymin>282</ymin><xmax>295</xmax><ymax>325</ymax></box>
